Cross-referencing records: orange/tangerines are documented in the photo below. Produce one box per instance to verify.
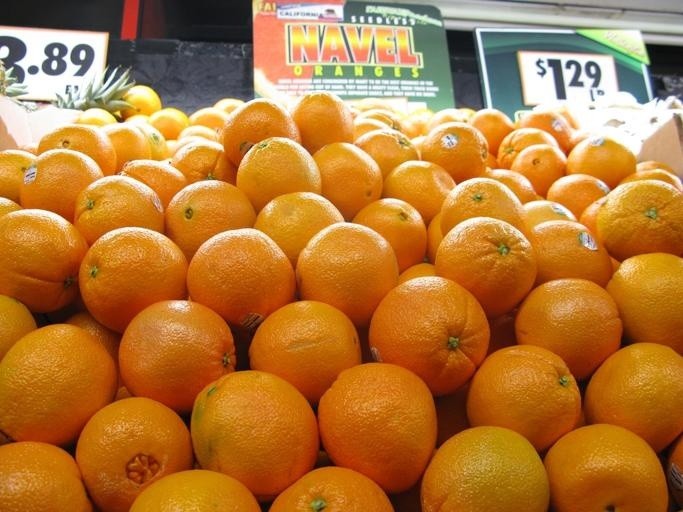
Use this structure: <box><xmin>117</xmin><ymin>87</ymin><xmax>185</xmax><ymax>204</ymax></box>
<box><xmin>0</xmin><ymin>84</ymin><xmax>683</xmax><ymax>512</ymax></box>
